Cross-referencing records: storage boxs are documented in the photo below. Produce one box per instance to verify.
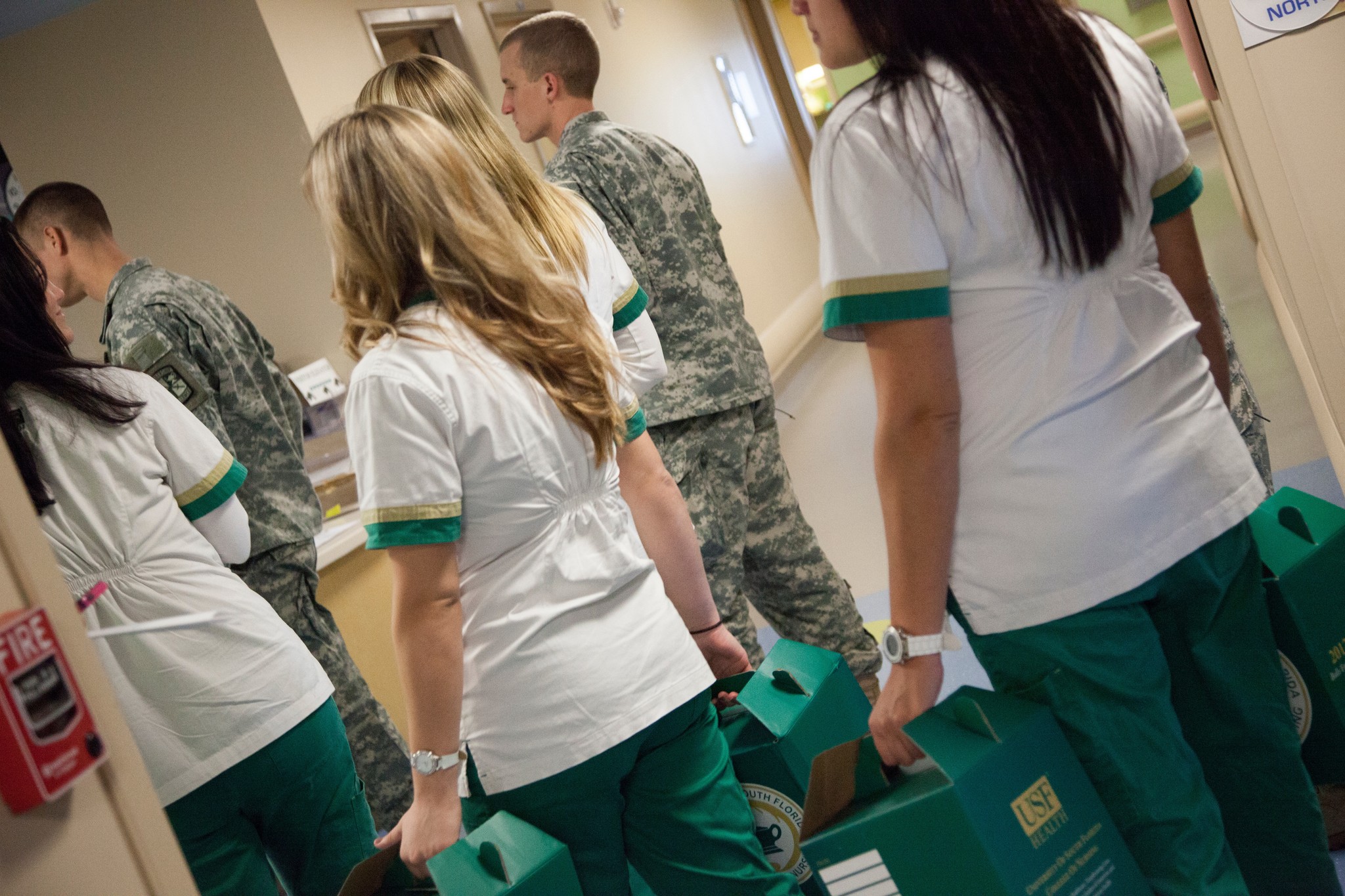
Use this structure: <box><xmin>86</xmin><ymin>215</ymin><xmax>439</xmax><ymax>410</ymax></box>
<box><xmin>1248</xmin><ymin>483</ymin><xmax>1345</xmax><ymax>788</ymax></box>
<box><xmin>794</xmin><ymin>685</ymin><xmax>1154</xmax><ymax>896</ymax></box>
<box><xmin>345</xmin><ymin>811</ymin><xmax>587</xmax><ymax>896</ymax></box>
<box><xmin>708</xmin><ymin>628</ymin><xmax>880</xmax><ymax>896</ymax></box>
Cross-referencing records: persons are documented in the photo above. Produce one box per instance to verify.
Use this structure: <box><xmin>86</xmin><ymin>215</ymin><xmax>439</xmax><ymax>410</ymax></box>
<box><xmin>12</xmin><ymin>181</ymin><xmax>414</xmax><ymax>839</ymax></box>
<box><xmin>352</xmin><ymin>53</ymin><xmax>668</xmax><ymax>409</ymax></box>
<box><xmin>298</xmin><ymin>103</ymin><xmax>802</xmax><ymax>896</ymax></box>
<box><xmin>1</xmin><ymin>216</ymin><xmax>382</xmax><ymax>896</ymax></box>
<box><xmin>791</xmin><ymin>0</ymin><xmax>1345</xmax><ymax>896</ymax></box>
<box><xmin>496</xmin><ymin>11</ymin><xmax>882</xmax><ymax>713</ymax></box>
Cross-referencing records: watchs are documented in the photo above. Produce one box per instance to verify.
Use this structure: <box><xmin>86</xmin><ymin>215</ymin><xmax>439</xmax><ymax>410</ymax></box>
<box><xmin>410</xmin><ymin>748</ymin><xmax>461</xmax><ymax>776</ymax></box>
<box><xmin>882</xmin><ymin>625</ymin><xmax>944</xmax><ymax>666</ymax></box>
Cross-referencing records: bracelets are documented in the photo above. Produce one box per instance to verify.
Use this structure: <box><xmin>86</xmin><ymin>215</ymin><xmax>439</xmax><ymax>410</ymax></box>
<box><xmin>690</xmin><ymin>619</ymin><xmax>723</xmax><ymax>635</ymax></box>
